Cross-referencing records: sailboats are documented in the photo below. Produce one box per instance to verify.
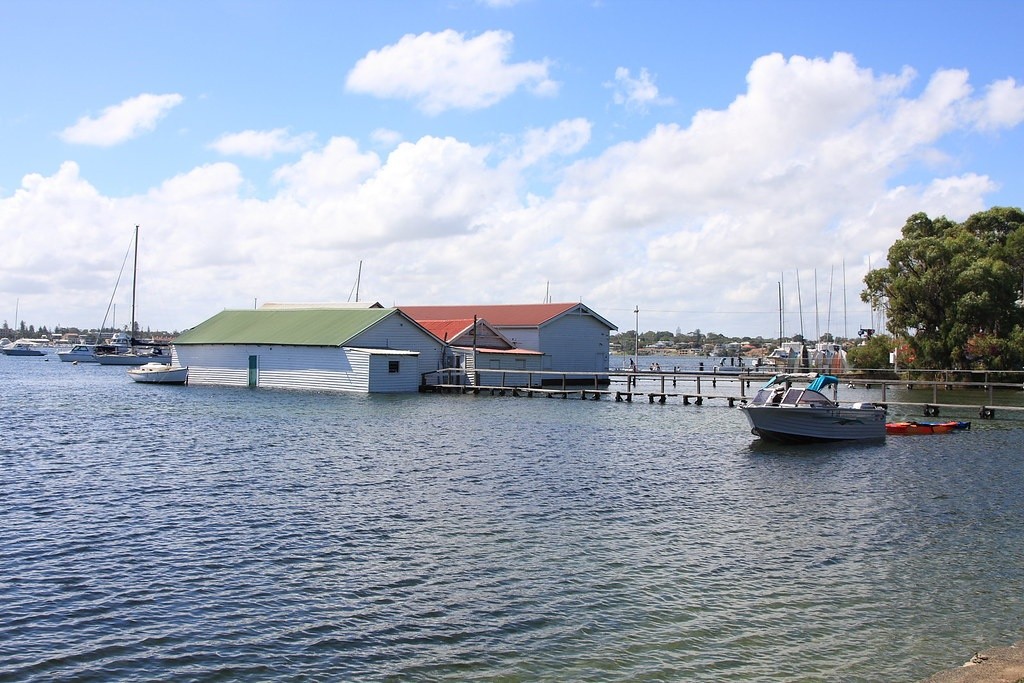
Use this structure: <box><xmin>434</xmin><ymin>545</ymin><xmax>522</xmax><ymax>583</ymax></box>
<box><xmin>92</xmin><ymin>224</ymin><xmax>174</xmax><ymax>365</ymax></box>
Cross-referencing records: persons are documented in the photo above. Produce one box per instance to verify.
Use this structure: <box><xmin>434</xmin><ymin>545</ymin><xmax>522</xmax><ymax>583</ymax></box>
<box><xmin>720</xmin><ymin>358</ymin><xmax>726</xmax><ymax>366</ymax></box>
<box><xmin>656</xmin><ymin>363</ymin><xmax>660</xmax><ymax>370</ymax></box>
<box><xmin>651</xmin><ymin>363</ymin><xmax>656</xmax><ymax>370</ymax></box>
<box><xmin>630</xmin><ymin>358</ymin><xmax>634</xmax><ymax>365</ymax></box>
<box><xmin>772</xmin><ymin>387</ymin><xmax>786</xmax><ymax>406</ymax></box>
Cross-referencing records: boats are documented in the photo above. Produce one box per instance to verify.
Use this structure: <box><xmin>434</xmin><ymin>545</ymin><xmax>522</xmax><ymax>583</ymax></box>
<box><xmin>59</xmin><ymin>344</ymin><xmax>127</xmax><ymax>362</ymax></box>
<box><xmin>735</xmin><ymin>373</ymin><xmax>889</xmax><ymax>443</ymax></box>
<box><xmin>911</xmin><ymin>419</ymin><xmax>972</xmax><ymax>433</ymax></box>
<box><xmin>127</xmin><ymin>361</ymin><xmax>190</xmax><ymax>383</ymax></box>
<box><xmin>884</xmin><ymin>419</ymin><xmax>958</xmax><ymax>436</ymax></box>
<box><xmin>2</xmin><ymin>340</ymin><xmax>47</xmax><ymax>356</ymax></box>
<box><xmin>107</xmin><ymin>333</ymin><xmax>132</xmax><ymax>348</ymax></box>
